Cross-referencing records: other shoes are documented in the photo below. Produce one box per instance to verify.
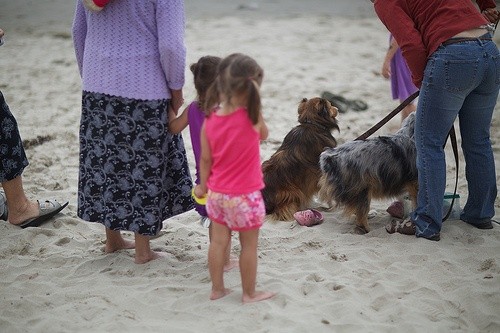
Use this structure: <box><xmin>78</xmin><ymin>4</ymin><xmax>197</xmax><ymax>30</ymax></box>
<box><xmin>293</xmin><ymin>208</ymin><xmax>324</xmax><ymax>226</ymax></box>
<box><xmin>397</xmin><ymin>218</ymin><xmax>440</xmax><ymax>241</ymax></box>
<box><xmin>321</xmin><ymin>90</ymin><xmax>367</xmax><ymax>112</ymax></box>
<box><xmin>474</xmin><ymin>221</ymin><xmax>493</xmax><ymax>229</ymax></box>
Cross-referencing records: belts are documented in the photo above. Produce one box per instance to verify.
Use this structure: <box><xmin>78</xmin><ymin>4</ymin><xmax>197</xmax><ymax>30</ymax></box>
<box><xmin>442</xmin><ymin>38</ymin><xmax>492</xmax><ymax>45</ymax></box>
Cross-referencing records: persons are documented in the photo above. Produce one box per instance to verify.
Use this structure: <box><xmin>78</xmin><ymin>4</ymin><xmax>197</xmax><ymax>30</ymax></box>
<box><xmin>370</xmin><ymin>0</ymin><xmax>500</xmax><ymax>240</ymax></box>
<box><xmin>194</xmin><ymin>52</ymin><xmax>275</xmax><ymax>302</ymax></box>
<box><xmin>0</xmin><ymin>1</ymin><xmax>239</xmax><ymax>272</ymax></box>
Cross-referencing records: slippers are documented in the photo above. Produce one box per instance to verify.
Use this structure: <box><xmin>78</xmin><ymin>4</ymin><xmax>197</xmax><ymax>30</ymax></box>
<box><xmin>20</xmin><ymin>199</ymin><xmax>69</xmax><ymax>229</ymax></box>
<box><xmin>0</xmin><ymin>191</ymin><xmax>9</xmax><ymax>221</ymax></box>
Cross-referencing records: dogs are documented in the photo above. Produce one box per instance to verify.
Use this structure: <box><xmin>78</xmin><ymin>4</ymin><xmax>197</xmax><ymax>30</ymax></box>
<box><xmin>319</xmin><ymin>112</ymin><xmax>415</xmax><ymax>235</ymax></box>
<box><xmin>261</xmin><ymin>97</ymin><xmax>341</xmax><ymax>221</ymax></box>
